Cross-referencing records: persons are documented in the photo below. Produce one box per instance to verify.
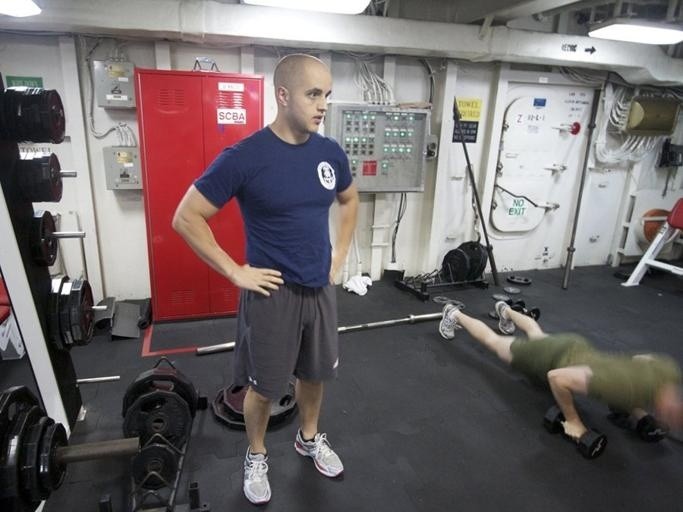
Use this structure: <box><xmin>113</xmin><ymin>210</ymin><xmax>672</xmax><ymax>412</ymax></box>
<box><xmin>437</xmin><ymin>301</ymin><xmax>683</xmax><ymax>446</ymax></box>
<box><xmin>171</xmin><ymin>52</ymin><xmax>360</xmax><ymax>505</ymax></box>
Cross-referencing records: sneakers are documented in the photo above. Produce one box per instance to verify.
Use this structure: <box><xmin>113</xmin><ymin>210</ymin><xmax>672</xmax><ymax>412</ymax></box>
<box><xmin>494</xmin><ymin>300</ymin><xmax>515</xmax><ymax>335</ymax></box>
<box><xmin>438</xmin><ymin>304</ymin><xmax>457</xmax><ymax>340</ymax></box>
<box><xmin>294</xmin><ymin>427</ymin><xmax>344</xmax><ymax>478</ymax></box>
<box><xmin>242</xmin><ymin>445</ymin><xmax>272</xmax><ymax>506</ymax></box>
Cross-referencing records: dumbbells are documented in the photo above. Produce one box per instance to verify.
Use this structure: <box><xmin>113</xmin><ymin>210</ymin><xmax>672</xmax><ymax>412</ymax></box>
<box><xmin>607</xmin><ymin>407</ymin><xmax>667</xmax><ymax>443</ymax></box>
<box><xmin>543</xmin><ymin>403</ymin><xmax>607</xmax><ymax>459</ymax></box>
<box><xmin>489</xmin><ymin>287</ymin><xmax>541</xmax><ymax>322</ymax></box>
